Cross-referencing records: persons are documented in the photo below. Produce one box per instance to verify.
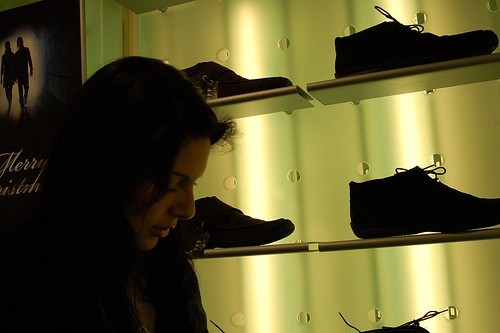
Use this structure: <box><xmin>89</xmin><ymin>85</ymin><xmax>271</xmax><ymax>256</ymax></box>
<box><xmin>0</xmin><ymin>55</ymin><xmax>233</xmax><ymax>332</ymax></box>
<box><xmin>0</xmin><ymin>36</ymin><xmax>33</xmax><ymax>113</ymax></box>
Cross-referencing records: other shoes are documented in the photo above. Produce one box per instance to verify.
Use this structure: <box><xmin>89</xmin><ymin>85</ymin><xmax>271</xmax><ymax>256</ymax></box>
<box><xmin>180</xmin><ymin>60</ymin><xmax>293</xmax><ymax>100</ymax></box>
<box><xmin>349</xmin><ymin>165</ymin><xmax>500</xmax><ymax>238</ymax></box>
<box><xmin>335</xmin><ymin>5</ymin><xmax>499</xmax><ymax>77</ymax></box>
<box><xmin>165</xmin><ymin>196</ymin><xmax>294</xmax><ymax>254</ymax></box>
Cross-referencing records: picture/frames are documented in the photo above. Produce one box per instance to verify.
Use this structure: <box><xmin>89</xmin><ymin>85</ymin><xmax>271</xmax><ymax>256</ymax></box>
<box><xmin>0</xmin><ymin>0</ymin><xmax>86</xmax><ymax>231</ymax></box>
